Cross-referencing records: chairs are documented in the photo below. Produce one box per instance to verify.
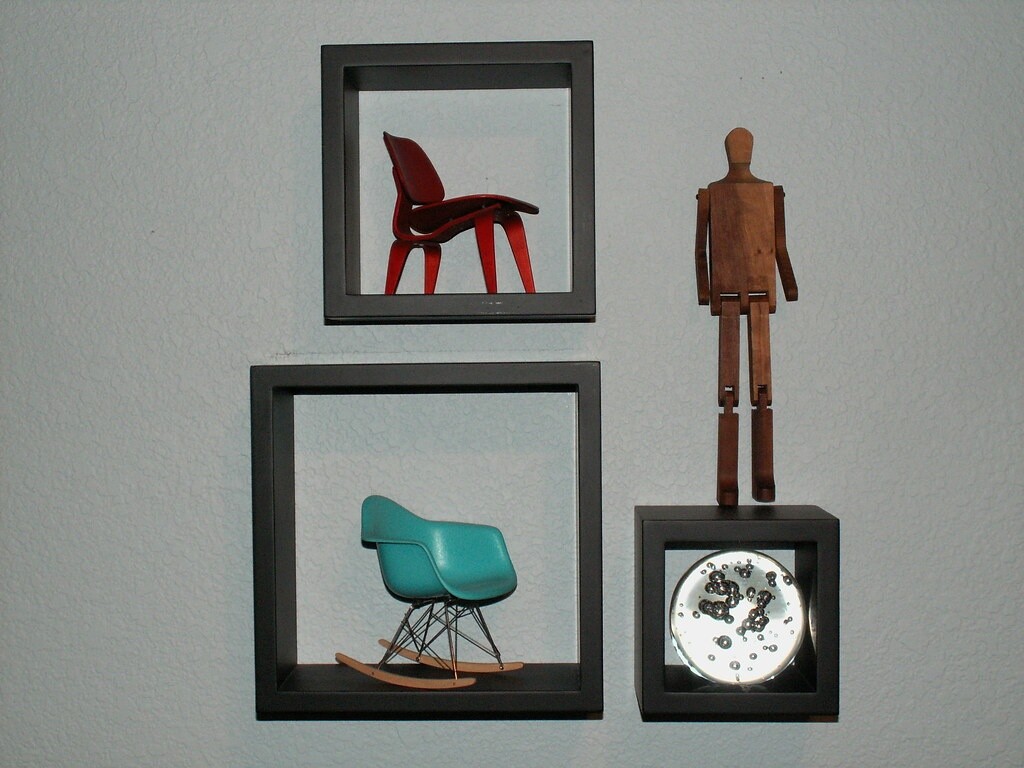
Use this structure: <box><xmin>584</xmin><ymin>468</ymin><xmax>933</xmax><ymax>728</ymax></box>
<box><xmin>382</xmin><ymin>125</ymin><xmax>540</xmax><ymax>295</ymax></box>
<box><xmin>335</xmin><ymin>487</ymin><xmax>532</xmax><ymax>690</ymax></box>
